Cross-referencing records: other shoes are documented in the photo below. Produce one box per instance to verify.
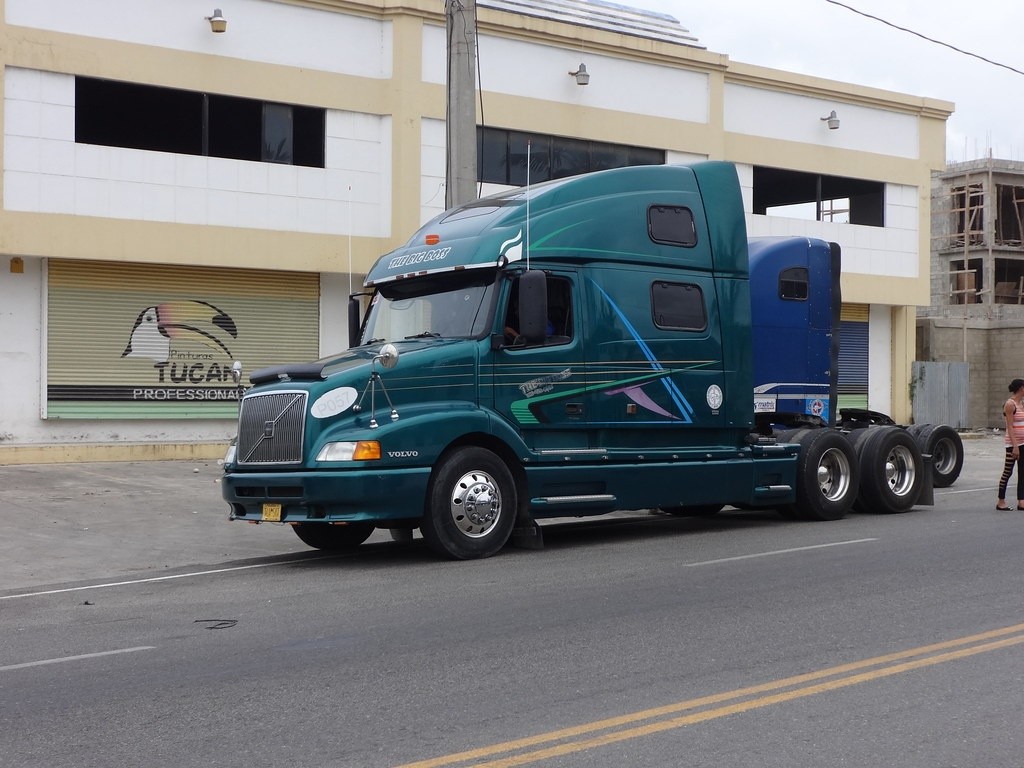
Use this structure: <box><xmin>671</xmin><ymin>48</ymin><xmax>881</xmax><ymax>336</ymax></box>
<box><xmin>996</xmin><ymin>505</ymin><xmax>1013</xmax><ymax>511</ymax></box>
<box><xmin>1018</xmin><ymin>505</ymin><xmax>1024</xmax><ymax>510</ymax></box>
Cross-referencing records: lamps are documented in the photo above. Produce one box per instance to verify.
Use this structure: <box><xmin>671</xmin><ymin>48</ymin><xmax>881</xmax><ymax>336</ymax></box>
<box><xmin>204</xmin><ymin>9</ymin><xmax>227</xmax><ymax>33</ymax></box>
<box><xmin>568</xmin><ymin>63</ymin><xmax>590</xmax><ymax>85</ymax></box>
<box><xmin>820</xmin><ymin>110</ymin><xmax>840</xmax><ymax>129</ymax></box>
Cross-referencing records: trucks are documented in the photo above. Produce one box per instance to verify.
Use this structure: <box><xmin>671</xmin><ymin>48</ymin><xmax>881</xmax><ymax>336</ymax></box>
<box><xmin>209</xmin><ymin>156</ymin><xmax>965</xmax><ymax>562</ymax></box>
<box><xmin>744</xmin><ymin>233</ymin><xmax>963</xmax><ymax>491</ymax></box>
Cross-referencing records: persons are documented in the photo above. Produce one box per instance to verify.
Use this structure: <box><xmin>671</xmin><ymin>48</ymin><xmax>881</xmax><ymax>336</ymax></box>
<box><xmin>995</xmin><ymin>379</ymin><xmax>1024</xmax><ymax>511</ymax></box>
<box><xmin>503</xmin><ymin>309</ymin><xmax>554</xmax><ymax>346</ymax></box>
